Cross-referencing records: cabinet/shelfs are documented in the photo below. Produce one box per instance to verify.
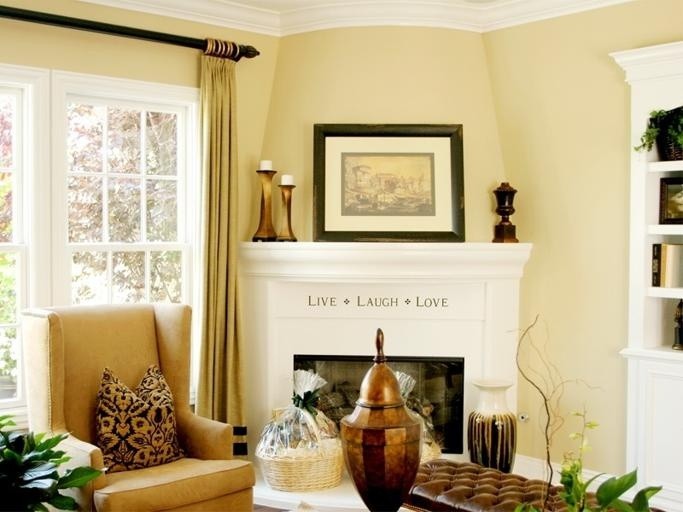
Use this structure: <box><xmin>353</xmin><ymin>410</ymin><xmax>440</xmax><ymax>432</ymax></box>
<box><xmin>237</xmin><ymin>242</ymin><xmax>532</xmax><ymax>466</ymax></box>
<box><xmin>606</xmin><ymin>42</ymin><xmax>682</xmax><ymax>511</ymax></box>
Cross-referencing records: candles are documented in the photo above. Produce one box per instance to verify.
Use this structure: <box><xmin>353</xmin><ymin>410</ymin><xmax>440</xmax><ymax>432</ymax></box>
<box><xmin>280</xmin><ymin>175</ymin><xmax>293</xmax><ymax>185</ymax></box>
<box><xmin>260</xmin><ymin>160</ymin><xmax>272</xmax><ymax>170</ymax></box>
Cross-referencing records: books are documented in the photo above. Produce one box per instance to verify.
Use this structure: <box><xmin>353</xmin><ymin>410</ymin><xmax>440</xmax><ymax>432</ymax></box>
<box><xmin>652</xmin><ymin>243</ymin><xmax>683</xmax><ymax>287</ymax></box>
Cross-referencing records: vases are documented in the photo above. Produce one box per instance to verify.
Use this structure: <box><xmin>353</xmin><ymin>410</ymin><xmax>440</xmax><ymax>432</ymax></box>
<box><xmin>467</xmin><ymin>376</ymin><xmax>518</xmax><ymax>474</ymax></box>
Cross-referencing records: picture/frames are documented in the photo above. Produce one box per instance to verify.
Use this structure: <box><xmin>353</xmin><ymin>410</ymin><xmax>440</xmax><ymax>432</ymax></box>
<box><xmin>312</xmin><ymin>123</ymin><xmax>465</xmax><ymax>242</ymax></box>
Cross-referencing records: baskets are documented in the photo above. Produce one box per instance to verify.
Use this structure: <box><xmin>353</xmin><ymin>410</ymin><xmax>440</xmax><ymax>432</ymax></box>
<box><xmin>256</xmin><ymin>408</ymin><xmax>346</xmax><ymax>493</ymax></box>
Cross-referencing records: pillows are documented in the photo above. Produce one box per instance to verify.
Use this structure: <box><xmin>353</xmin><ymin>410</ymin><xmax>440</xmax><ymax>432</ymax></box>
<box><xmin>96</xmin><ymin>366</ymin><xmax>185</xmax><ymax>473</ymax></box>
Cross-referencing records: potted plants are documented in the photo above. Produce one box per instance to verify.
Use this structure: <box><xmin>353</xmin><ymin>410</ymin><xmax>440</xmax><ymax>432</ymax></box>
<box><xmin>632</xmin><ymin>106</ymin><xmax>682</xmax><ymax>161</ymax></box>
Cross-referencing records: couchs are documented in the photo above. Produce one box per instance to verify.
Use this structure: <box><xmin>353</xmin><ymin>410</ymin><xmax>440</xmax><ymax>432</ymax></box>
<box><xmin>21</xmin><ymin>305</ymin><xmax>253</xmax><ymax>512</ymax></box>
<box><xmin>402</xmin><ymin>459</ymin><xmax>663</xmax><ymax>512</ymax></box>
